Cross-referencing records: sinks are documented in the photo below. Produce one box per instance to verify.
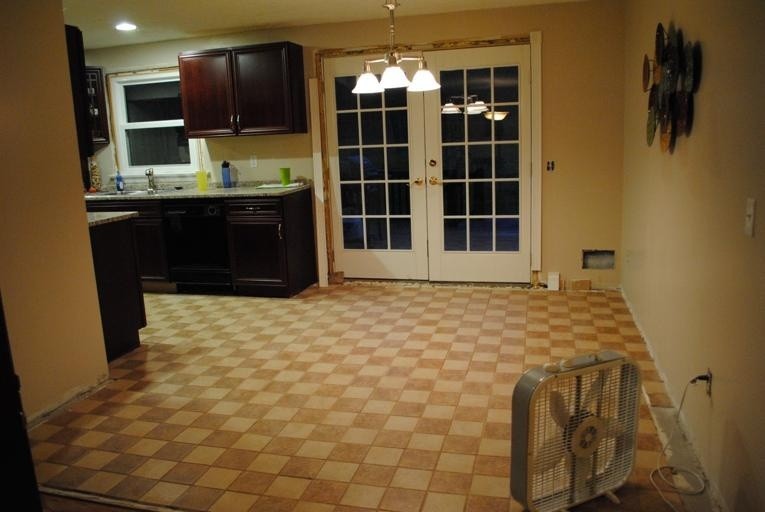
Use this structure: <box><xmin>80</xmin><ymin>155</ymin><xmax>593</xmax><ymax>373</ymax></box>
<box><xmin>100</xmin><ymin>191</ymin><xmax>137</xmax><ymax>195</ymax></box>
<box><xmin>131</xmin><ymin>190</ymin><xmax>174</xmax><ymax>194</ymax></box>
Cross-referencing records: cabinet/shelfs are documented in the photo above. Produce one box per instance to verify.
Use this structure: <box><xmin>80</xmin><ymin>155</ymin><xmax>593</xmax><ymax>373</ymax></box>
<box><xmin>84</xmin><ymin>194</ymin><xmax>166</xmax><ymax>293</ymax></box>
<box><xmin>86</xmin><ymin>211</ymin><xmax>147</xmax><ymax>363</ymax></box>
<box><xmin>225</xmin><ymin>182</ymin><xmax>317</xmax><ymax>298</ymax></box>
<box><xmin>85</xmin><ymin>64</ymin><xmax>111</xmax><ymax>148</ymax></box>
<box><xmin>62</xmin><ymin>21</ymin><xmax>97</xmax><ymax>158</ymax></box>
<box><xmin>177</xmin><ymin>40</ymin><xmax>312</xmax><ymax>140</ymax></box>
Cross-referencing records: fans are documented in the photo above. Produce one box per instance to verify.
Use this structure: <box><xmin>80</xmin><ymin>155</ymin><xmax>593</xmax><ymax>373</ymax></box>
<box><xmin>511</xmin><ymin>350</ymin><xmax>639</xmax><ymax>512</ymax></box>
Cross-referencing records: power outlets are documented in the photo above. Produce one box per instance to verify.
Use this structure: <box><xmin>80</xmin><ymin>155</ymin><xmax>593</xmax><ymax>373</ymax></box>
<box><xmin>704</xmin><ymin>368</ymin><xmax>713</xmax><ymax>397</ymax></box>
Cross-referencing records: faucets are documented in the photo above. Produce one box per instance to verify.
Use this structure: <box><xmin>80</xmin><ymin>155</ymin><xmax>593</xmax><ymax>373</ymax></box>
<box><xmin>145</xmin><ymin>168</ymin><xmax>155</xmax><ymax>190</ymax></box>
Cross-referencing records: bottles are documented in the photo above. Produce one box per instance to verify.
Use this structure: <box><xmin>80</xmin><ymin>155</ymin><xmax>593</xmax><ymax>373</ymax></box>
<box><xmin>88</xmin><ymin>152</ymin><xmax>103</xmax><ymax>191</ymax></box>
<box><xmin>115</xmin><ymin>169</ymin><xmax>125</xmax><ymax>193</ymax></box>
<box><xmin>220</xmin><ymin>159</ymin><xmax>232</xmax><ymax>188</ymax></box>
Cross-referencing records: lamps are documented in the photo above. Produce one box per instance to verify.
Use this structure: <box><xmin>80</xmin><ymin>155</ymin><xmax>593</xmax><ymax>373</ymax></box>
<box><xmin>349</xmin><ymin>0</ymin><xmax>441</xmax><ymax>97</ymax></box>
<box><xmin>441</xmin><ymin>93</ymin><xmax>510</xmax><ymax>121</ymax></box>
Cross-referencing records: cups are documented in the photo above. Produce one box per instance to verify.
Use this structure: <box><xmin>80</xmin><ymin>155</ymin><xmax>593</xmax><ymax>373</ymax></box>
<box><xmin>278</xmin><ymin>164</ymin><xmax>291</xmax><ymax>186</ymax></box>
<box><xmin>195</xmin><ymin>169</ymin><xmax>212</xmax><ymax>197</ymax></box>
